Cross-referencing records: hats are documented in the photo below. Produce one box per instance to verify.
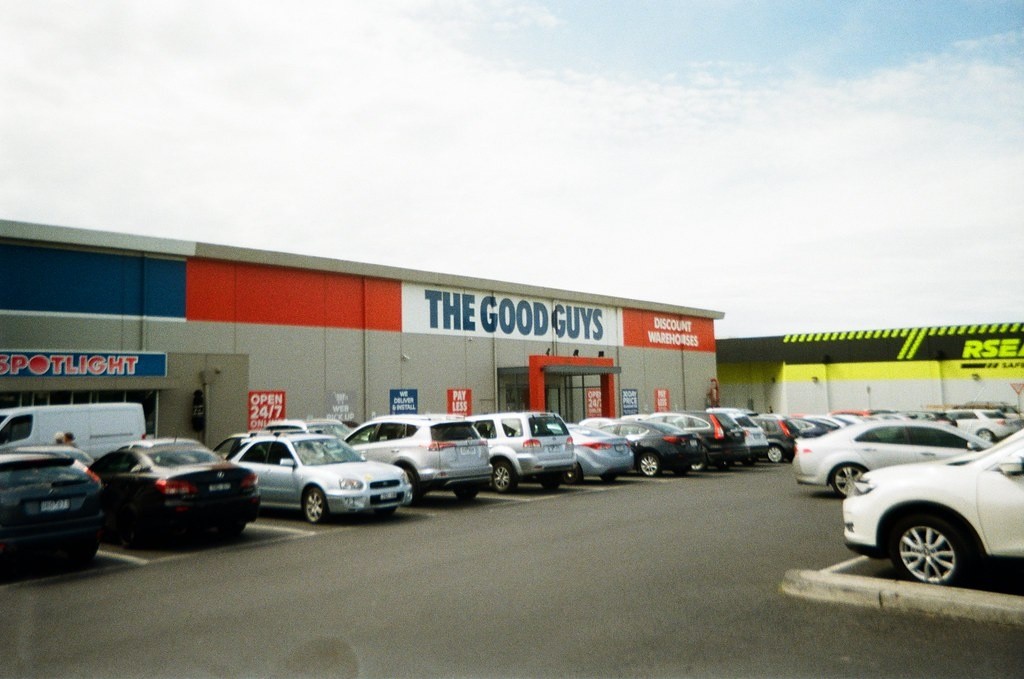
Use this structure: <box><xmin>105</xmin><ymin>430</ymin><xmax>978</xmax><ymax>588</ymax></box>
<box><xmin>56</xmin><ymin>432</ymin><xmax>63</xmax><ymax>440</ymax></box>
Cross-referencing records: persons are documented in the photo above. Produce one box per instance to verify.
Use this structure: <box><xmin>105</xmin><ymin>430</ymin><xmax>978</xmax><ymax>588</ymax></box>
<box><xmin>55</xmin><ymin>431</ymin><xmax>79</xmax><ymax>448</ymax></box>
<box><xmin>767</xmin><ymin>405</ymin><xmax>773</xmax><ymax>414</ymax></box>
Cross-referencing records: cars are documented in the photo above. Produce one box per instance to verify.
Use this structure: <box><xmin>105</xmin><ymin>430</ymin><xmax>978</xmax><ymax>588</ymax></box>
<box><xmin>0</xmin><ymin>453</ymin><xmax>106</xmax><ymax>580</ymax></box>
<box><xmin>214</xmin><ymin>418</ymin><xmax>388</xmax><ymax>460</ymax></box>
<box><xmin>644</xmin><ymin>411</ymin><xmax>749</xmax><ymax>471</ymax></box>
<box><xmin>546</xmin><ymin>423</ymin><xmax>634</xmax><ymax>484</ymax></box>
<box><xmin>597</xmin><ymin>421</ymin><xmax>706</xmax><ymax>477</ymax></box>
<box><xmin>120</xmin><ymin>438</ymin><xmax>203</xmax><ymax>448</ymax></box>
<box><xmin>464</xmin><ymin>411</ymin><xmax>577</xmax><ymax>494</ymax></box>
<box><xmin>751</xmin><ymin>413</ymin><xmax>798</xmax><ymax>464</ymax></box>
<box><xmin>88</xmin><ymin>448</ymin><xmax>260</xmax><ymax>549</ymax></box>
<box><xmin>900</xmin><ymin>411</ymin><xmax>958</xmax><ymax>429</ymax></box>
<box><xmin>703</xmin><ymin>408</ymin><xmax>768</xmax><ymax>466</ymax></box>
<box><xmin>790</xmin><ymin>410</ymin><xmax>910</xmax><ymax>438</ymax></box>
<box><xmin>228</xmin><ymin>434</ymin><xmax>412</xmax><ymax>525</ymax></box>
<box><xmin>578</xmin><ymin>414</ymin><xmax>650</xmax><ymax>429</ymax></box>
<box><xmin>0</xmin><ymin>445</ymin><xmax>94</xmax><ymax>468</ymax></box>
<box><xmin>344</xmin><ymin>414</ymin><xmax>492</xmax><ymax>506</ymax></box>
<box><xmin>944</xmin><ymin>409</ymin><xmax>1019</xmax><ymax>445</ymax></box>
<box><xmin>843</xmin><ymin>428</ymin><xmax>1024</xmax><ymax>586</ymax></box>
<box><xmin>793</xmin><ymin>420</ymin><xmax>995</xmax><ymax>500</ymax></box>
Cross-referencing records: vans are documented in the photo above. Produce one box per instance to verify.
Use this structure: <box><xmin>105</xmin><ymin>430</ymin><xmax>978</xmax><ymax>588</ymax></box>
<box><xmin>0</xmin><ymin>403</ymin><xmax>148</xmax><ymax>462</ymax></box>
